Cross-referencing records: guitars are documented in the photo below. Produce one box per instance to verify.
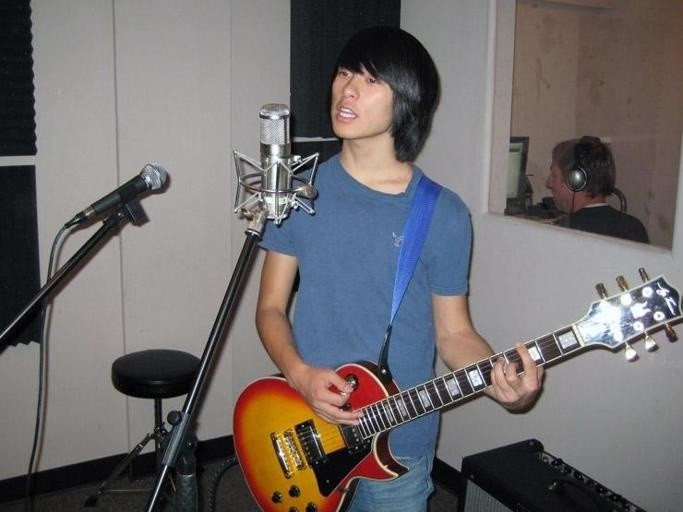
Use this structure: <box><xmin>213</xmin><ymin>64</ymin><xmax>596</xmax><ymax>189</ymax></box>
<box><xmin>232</xmin><ymin>268</ymin><xmax>682</xmax><ymax>511</ymax></box>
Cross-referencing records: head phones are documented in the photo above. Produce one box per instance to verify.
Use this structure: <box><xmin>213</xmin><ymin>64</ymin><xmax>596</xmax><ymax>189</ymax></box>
<box><xmin>565</xmin><ymin>135</ymin><xmax>600</xmax><ymax>193</ymax></box>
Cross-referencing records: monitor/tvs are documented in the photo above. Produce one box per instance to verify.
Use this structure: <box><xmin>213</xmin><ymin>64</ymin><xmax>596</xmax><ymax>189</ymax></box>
<box><xmin>506</xmin><ymin>136</ymin><xmax>530</xmax><ymax>205</ymax></box>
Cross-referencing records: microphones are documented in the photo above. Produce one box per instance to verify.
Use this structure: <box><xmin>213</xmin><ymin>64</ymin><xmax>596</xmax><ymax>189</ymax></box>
<box><xmin>260</xmin><ymin>104</ymin><xmax>291</xmax><ymax>218</ymax></box>
<box><xmin>61</xmin><ymin>164</ymin><xmax>171</xmax><ymax>229</ymax></box>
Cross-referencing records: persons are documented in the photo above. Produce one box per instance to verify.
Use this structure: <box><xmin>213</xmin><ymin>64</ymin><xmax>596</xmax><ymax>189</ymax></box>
<box><xmin>256</xmin><ymin>27</ymin><xmax>545</xmax><ymax>512</ymax></box>
<box><xmin>545</xmin><ymin>135</ymin><xmax>650</xmax><ymax>243</ymax></box>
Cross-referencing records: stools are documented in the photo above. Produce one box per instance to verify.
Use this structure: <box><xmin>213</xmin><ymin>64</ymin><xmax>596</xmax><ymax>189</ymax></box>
<box><xmin>80</xmin><ymin>348</ymin><xmax>228</xmax><ymax>510</ymax></box>
